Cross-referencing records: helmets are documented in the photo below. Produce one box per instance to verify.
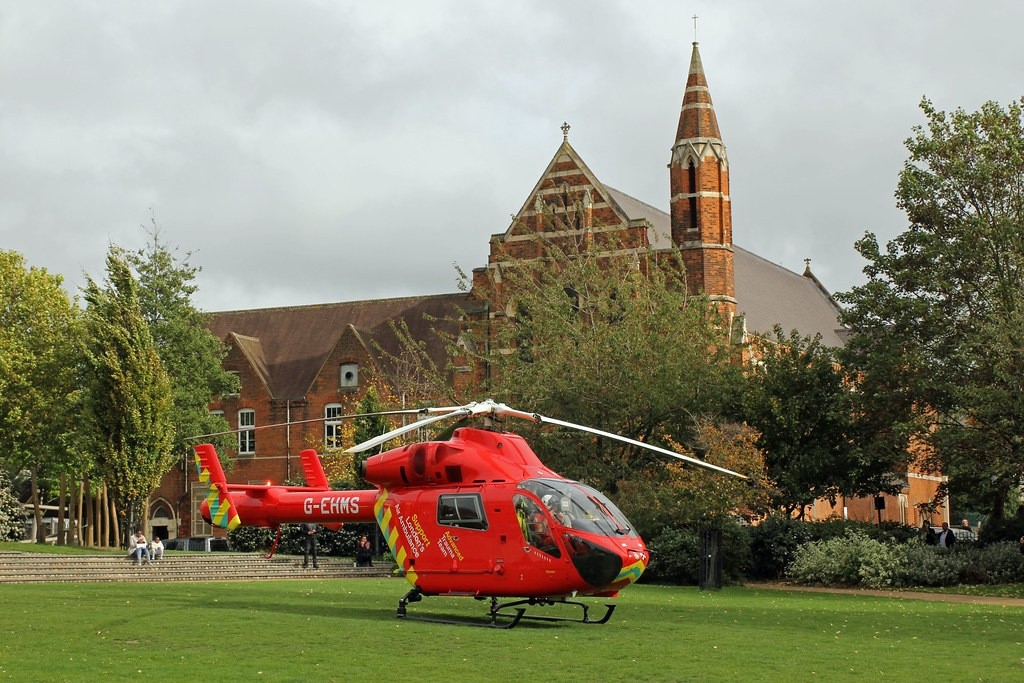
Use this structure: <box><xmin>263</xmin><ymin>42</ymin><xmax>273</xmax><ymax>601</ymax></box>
<box><xmin>539</xmin><ymin>495</ymin><xmax>558</xmax><ymax>510</ymax></box>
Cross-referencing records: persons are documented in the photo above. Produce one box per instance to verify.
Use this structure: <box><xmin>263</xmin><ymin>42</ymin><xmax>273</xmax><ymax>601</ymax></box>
<box><xmin>921</xmin><ymin>519</ymin><xmax>937</xmax><ymax>546</ymax></box>
<box><xmin>962</xmin><ymin>518</ymin><xmax>971</xmax><ymax>530</ymax></box>
<box><xmin>128</xmin><ymin>529</ymin><xmax>153</xmax><ymax>566</ymax></box>
<box><xmin>356</xmin><ymin>535</ymin><xmax>373</xmax><ymax>567</ymax></box>
<box><xmin>938</xmin><ymin>522</ymin><xmax>956</xmax><ymax>547</ymax></box>
<box><xmin>300</xmin><ymin>523</ymin><xmax>321</xmax><ymax>568</ymax></box>
<box><xmin>150</xmin><ymin>537</ymin><xmax>164</xmax><ymax>560</ymax></box>
<box><xmin>532</xmin><ymin>493</ymin><xmax>579</xmax><ymax>557</ymax></box>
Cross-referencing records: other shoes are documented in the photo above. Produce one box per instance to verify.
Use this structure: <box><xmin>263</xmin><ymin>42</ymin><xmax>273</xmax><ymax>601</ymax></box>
<box><xmin>357</xmin><ymin>564</ymin><xmax>362</xmax><ymax>567</ymax></box>
<box><xmin>145</xmin><ymin>562</ymin><xmax>152</xmax><ymax>565</ymax></box>
<box><xmin>158</xmin><ymin>557</ymin><xmax>163</xmax><ymax>560</ymax></box>
<box><xmin>313</xmin><ymin>564</ymin><xmax>319</xmax><ymax>568</ymax></box>
<box><xmin>135</xmin><ymin>563</ymin><xmax>141</xmax><ymax>566</ymax></box>
<box><xmin>152</xmin><ymin>558</ymin><xmax>155</xmax><ymax>560</ymax></box>
<box><xmin>303</xmin><ymin>564</ymin><xmax>308</xmax><ymax>567</ymax></box>
<box><xmin>369</xmin><ymin>565</ymin><xmax>374</xmax><ymax>567</ymax></box>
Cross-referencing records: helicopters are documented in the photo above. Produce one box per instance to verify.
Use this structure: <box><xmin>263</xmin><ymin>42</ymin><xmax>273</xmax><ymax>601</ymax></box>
<box><xmin>185</xmin><ymin>399</ymin><xmax>748</xmax><ymax>630</ymax></box>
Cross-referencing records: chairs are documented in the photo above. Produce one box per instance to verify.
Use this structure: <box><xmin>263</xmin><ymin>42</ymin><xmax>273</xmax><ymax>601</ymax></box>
<box><xmin>516</xmin><ymin>498</ymin><xmax>556</xmax><ymax>552</ymax></box>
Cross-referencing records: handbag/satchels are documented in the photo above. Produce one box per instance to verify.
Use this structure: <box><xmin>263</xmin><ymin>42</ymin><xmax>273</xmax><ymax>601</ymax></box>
<box><xmin>136</xmin><ymin>542</ymin><xmax>146</xmax><ymax>548</ymax></box>
